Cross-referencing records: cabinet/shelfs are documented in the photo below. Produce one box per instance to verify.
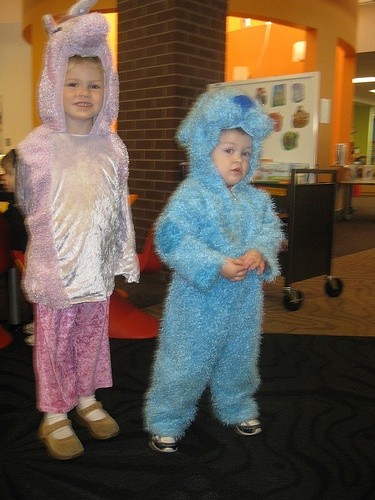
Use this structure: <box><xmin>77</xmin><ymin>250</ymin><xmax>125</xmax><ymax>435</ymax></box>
<box><xmin>246</xmin><ymin>170</ymin><xmax>344</xmax><ymax>310</ymax></box>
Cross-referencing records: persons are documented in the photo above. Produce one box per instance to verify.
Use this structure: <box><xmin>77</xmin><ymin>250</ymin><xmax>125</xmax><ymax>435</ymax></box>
<box><xmin>0</xmin><ymin>148</ymin><xmax>34</xmax><ymax>345</ymax></box>
<box><xmin>14</xmin><ymin>53</ymin><xmax>141</xmax><ymax>460</ymax></box>
<box><xmin>145</xmin><ymin>123</ymin><xmax>284</xmax><ymax>453</ymax></box>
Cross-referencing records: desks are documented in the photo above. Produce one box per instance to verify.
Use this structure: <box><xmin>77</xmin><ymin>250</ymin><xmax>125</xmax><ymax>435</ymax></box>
<box><xmin>337</xmin><ymin>181</ymin><xmax>375</xmax><ymax>219</ymax></box>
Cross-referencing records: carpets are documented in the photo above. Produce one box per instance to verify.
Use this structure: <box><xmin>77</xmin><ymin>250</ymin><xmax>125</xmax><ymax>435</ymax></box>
<box><xmin>0</xmin><ymin>333</ymin><xmax>375</xmax><ymax>500</ymax></box>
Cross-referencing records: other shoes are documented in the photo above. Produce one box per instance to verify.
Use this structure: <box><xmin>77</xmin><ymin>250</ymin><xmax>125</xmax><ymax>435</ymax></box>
<box><xmin>71</xmin><ymin>401</ymin><xmax>120</xmax><ymax>440</ymax></box>
<box><xmin>23</xmin><ymin>322</ymin><xmax>35</xmax><ymax>334</ymax></box>
<box><xmin>24</xmin><ymin>335</ymin><xmax>34</xmax><ymax>346</ymax></box>
<box><xmin>234</xmin><ymin>419</ymin><xmax>262</xmax><ymax>435</ymax></box>
<box><xmin>36</xmin><ymin>413</ymin><xmax>85</xmax><ymax>461</ymax></box>
<box><xmin>149</xmin><ymin>432</ymin><xmax>178</xmax><ymax>453</ymax></box>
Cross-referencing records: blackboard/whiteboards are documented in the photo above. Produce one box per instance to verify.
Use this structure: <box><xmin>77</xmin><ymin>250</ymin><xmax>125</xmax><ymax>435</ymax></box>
<box><xmin>209</xmin><ymin>72</ymin><xmax>321</xmax><ymax>185</ymax></box>
